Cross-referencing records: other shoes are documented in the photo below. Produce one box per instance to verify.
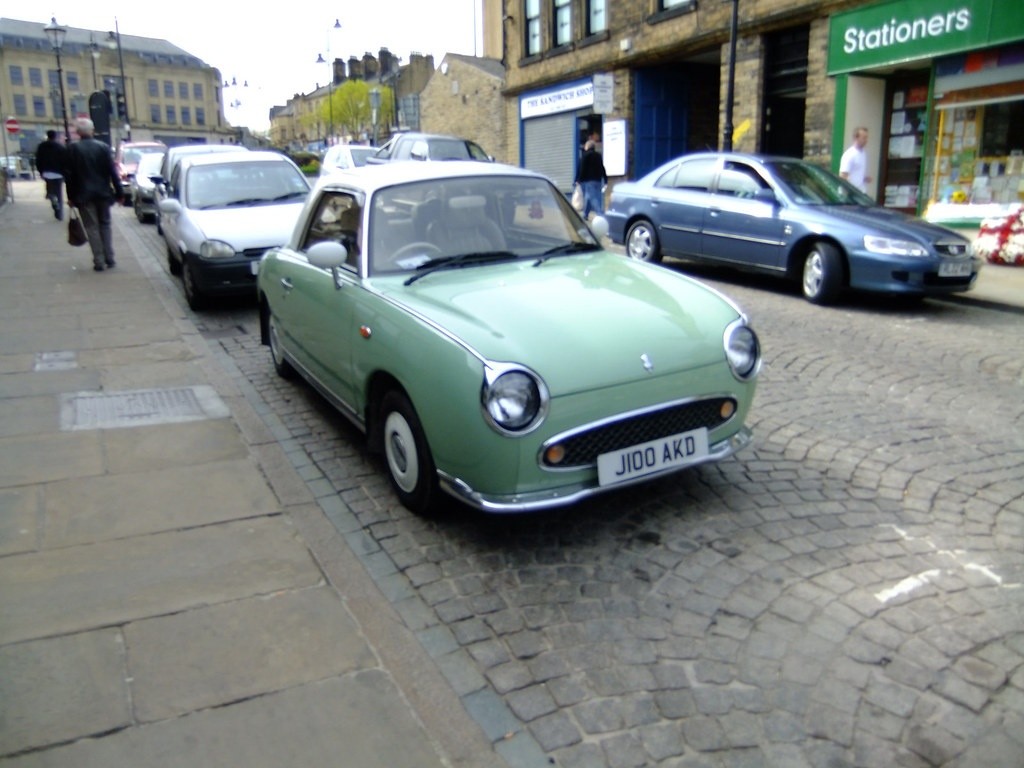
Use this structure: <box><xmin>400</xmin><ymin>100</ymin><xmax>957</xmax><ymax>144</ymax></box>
<box><xmin>107</xmin><ymin>257</ymin><xmax>114</xmax><ymax>267</ymax></box>
<box><xmin>52</xmin><ymin>196</ymin><xmax>62</xmax><ymax>220</ymax></box>
<box><xmin>94</xmin><ymin>266</ymin><xmax>107</xmax><ymax>271</ymax></box>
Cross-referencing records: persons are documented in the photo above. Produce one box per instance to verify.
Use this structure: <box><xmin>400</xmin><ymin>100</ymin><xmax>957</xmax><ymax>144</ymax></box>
<box><xmin>35</xmin><ymin>129</ymin><xmax>68</xmax><ymax>221</ymax></box>
<box><xmin>572</xmin><ymin>141</ymin><xmax>609</xmax><ymax>222</ymax></box>
<box><xmin>838</xmin><ymin>126</ymin><xmax>872</xmax><ymax>200</ymax></box>
<box><xmin>63</xmin><ymin>117</ymin><xmax>126</xmax><ymax>272</ymax></box>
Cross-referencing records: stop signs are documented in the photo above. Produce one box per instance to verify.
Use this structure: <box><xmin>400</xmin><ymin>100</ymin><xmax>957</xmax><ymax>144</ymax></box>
<box><xmin>6</xmin><ymin>119</ymin><xmax>19</xmax><ymax>133</ymax></box>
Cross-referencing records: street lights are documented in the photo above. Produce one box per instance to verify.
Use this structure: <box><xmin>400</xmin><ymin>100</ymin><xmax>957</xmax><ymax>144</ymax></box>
<box><xmin>89</xmin><ymin>31</ymin><xmax>101</xmax><ymax>92</ymax></box>
<box><xmin>44</xmin><ymin>17</ymin><xmax>71</xmax><ymax>146</ymax></box>
<box><xmin>367</xmin><ymin>87</ymin><xmax>384</xmax><ymax>147</ymax></box>
<box><xmin>315</xmin><ymin>19</ymin><xmax>342</xmax><ymax>146</ymax></box>
<box><xmin>105</xmin><ymin>17</ymin><xmax>131</xmax><ymax>141</ymax></box>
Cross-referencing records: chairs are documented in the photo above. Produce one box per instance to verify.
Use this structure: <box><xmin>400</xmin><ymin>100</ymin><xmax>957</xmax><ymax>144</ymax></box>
<box><xmin>340</xmin><ymin>207</ymin><xmax>392</xmax><ymax>274</ymax></box>
<box><xmin>410</xmin><ymin>196</ymin><xmax>507</xmax><ymax>257</ymax></box>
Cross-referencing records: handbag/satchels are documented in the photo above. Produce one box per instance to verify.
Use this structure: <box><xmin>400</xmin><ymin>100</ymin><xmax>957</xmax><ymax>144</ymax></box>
<box><xmin>67</xmin><ymin>207</ymin><xmax>86</xmax><ymax>246</ymax></box>
<box><xmin>571</xmin><ymin>183</ymin><xmax>583</xmax><ymax>211</ymax></box>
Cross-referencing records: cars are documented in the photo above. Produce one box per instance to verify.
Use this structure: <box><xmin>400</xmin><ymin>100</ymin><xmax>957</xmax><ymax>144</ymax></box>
<box><xmin>256</xmin><ymin>161</ymin><xmax>762</xmax><ymax>516</ymax></box>
<box><xmin>604</xmin><ymin>152</ymin><xmax>983</xmax><ymax>306</ymax></box>
<box><xmin>317</xmin><ymin>134</ymin><xmax>514</xmax><ymax>226</ymax></box>
<box><xmin>110</xmin><ymin>140</ymin><xmax>349</xmax><ymax>311</ymax></box>
<box><xmin>0</xmin><ymin>156</ymin><xmax>22</xmax><ymax>178</ymax></box>
